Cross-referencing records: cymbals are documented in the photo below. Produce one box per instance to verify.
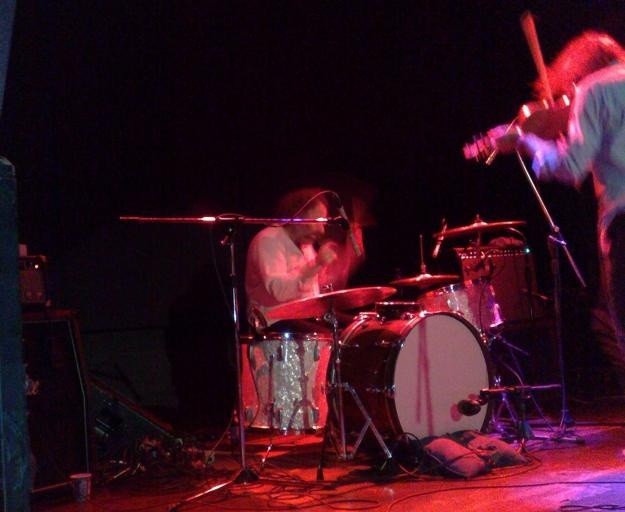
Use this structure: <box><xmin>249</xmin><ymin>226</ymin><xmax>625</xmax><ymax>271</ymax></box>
<box><xmin>266</xmin><ymin>286</ymin><xmax>398</xmax><ymax>320</ymax></box>
<box><xmin>433</xmin><ymin>221</ymin><xmax>527</xmax><ymax>238</ymax></box>
<box><xmin>389</xmin><ymin>274</ymin><xmax>460</xmax><ymax>288</ymax></box>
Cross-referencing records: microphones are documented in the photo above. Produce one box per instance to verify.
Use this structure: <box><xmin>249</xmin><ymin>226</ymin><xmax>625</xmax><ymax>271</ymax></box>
<box><xmin>335</xmin><ymin>205</ymin><xmax>362</xmax><ymax>256</ymax></box>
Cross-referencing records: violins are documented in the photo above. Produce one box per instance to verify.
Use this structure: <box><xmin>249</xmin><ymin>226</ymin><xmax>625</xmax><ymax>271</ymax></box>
<box><xmin>461</xmin><ymin>91</ymin><xmax>572</xmax><ymax>161</ymax></box>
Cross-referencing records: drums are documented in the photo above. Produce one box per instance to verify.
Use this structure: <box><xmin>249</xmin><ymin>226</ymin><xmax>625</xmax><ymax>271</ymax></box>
<box><xmin>325</xmin><ymin>310</ymin><xmax>495</xmax><ymax>468</ymax></box>
<box><xmin>234</xmin><ymin>331</ymin><xmax>334</xmax><ymax>437</ymax></box>
<box><xmin>415</xmin><ymin>275</ymin><xmax>504</xmax><ymax>333</ymax></box>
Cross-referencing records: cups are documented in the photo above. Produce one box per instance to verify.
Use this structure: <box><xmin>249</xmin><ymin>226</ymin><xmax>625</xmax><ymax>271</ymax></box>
<box><xmin>69</xmin><ymin>472</ymin><xmax>93</xmax><ymax>504</ymax></box>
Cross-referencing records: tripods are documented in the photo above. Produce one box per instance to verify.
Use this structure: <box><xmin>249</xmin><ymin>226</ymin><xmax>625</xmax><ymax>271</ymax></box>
<box><xmin>118</xmin><ymin>213</ymin><xmax>336</xmax><ymax>512</ymax></box>
<box><xmin>528</xmin><ymin>119</ymin><xmax>625</xmax><ymax>442</ymax></box>
<box><xmin>257</xmin><ymin>324</ymin><xmax>393</xmax><ymax>481</ymax></box>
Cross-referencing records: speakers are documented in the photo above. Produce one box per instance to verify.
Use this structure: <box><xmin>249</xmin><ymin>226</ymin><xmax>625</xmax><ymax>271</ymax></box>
<box><xmin>88</xmin><ymin>373</ymin><xmax>174</xmax><ymax>486</ymax></box>
<box><xmin>17</xmin><ymin>320</ymin><xmax>91</xmax><ymax>494</ymax></box>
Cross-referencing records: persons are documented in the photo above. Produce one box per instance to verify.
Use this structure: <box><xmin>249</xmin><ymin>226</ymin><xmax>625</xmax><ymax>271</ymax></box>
<box><xmin>244</xmin><ymin>188</ymin><xmax>371</xmax><ymax>461</ymax></box>
<box><xmin>491</xmin><ymin>31</ymin><xmax>625</xmax><ymax>354</ymax></box>
